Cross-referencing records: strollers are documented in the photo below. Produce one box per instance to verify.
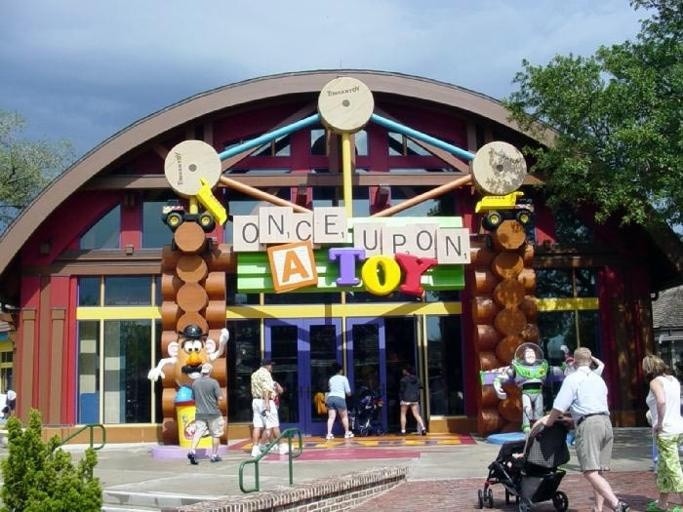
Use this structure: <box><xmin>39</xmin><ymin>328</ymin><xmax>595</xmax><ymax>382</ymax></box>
<box><xmin>477</xmin><ymin>412</ymin><xmax>573</xmax><ymax>512</ymax></box>
<box><xmin>346</xmin><ymin>380</ymin><xmax>384</xmax><ymax>437</ymax></box>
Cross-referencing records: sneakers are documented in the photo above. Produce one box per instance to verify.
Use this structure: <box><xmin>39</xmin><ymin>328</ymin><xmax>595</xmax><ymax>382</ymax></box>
<box><xmin>251</xmin><ymin>449</ymin><xmax>261</xmax><ymax>456</ymax></box>
<box><xmin>344</xmin><ymin>431</ymin><xmax>354</xmax><ymax>439</ymax></box>
<box><xmin>615</xmin><ymin>500</ymin><xmax>630</xmax><ymax>512</ymax></box>
<box><xmin>280</xmin><ymin>446</ymin><xmax>294</xmax><ymax>453</ymax></box>
<box><xmin>325</xmin><ymin>434</ymin><xmax>334</xmax><ymax>439</ymax></box>
<box><xmin>187</xmin><ymin>452</ymin><xmax>198</xmax><ymax>465</ymax></box>
<box><xmin>211</xmin><ymin>455</ymin><xmax>222</xmax><ymax>462</ymax></box>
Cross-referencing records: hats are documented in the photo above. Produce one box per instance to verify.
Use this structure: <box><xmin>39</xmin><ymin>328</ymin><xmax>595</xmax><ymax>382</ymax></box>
<box><xmin>201</xmin><ymin>364</ymin><xmax>213</xmax><ymax>373</ymax></box>
<box><xmin>261</xmin><ymin>358</ymin><xmax>275</xmax><ymax>365</ymax></box>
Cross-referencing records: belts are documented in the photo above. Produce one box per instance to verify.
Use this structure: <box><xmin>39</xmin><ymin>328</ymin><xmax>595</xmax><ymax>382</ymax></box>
<box><xmin>576</xmin><ymin>413</ymin><xmax>603</xmax><ymax>425</ymax></box>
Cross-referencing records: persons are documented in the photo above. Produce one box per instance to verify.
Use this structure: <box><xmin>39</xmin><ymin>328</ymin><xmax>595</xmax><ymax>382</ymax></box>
<box><xmin>641</xmin><ymin>354</ymin><xmax>683</xmax><ymax>511</ymax></box>
<box><xmin>249</xmin><ymin>358</ymin><xmax>294</xmax><ymax>457</ymax></box>
<box><xmin>540</xmin><ymin>347</ymin><xmax>630</xmax><ymax>512</ymax></box>
<box><xmin>493</xmin><ymin>341</ymin><xmax>571</xmax><ymax>433</ymax></box>
<box><xmin>396</xmin><ymin>365</ymin><xmax>426</xmax><ymax>436</ymax></box>
<box><xmin>324</xmin><ymin>366</ymin><xmax>355</xmax><ymax>439</ymax></box>
<box><xmin>187</xmin><ymin>363</ymin><xmax>225</xmax><ymax>465</ymax></box>
